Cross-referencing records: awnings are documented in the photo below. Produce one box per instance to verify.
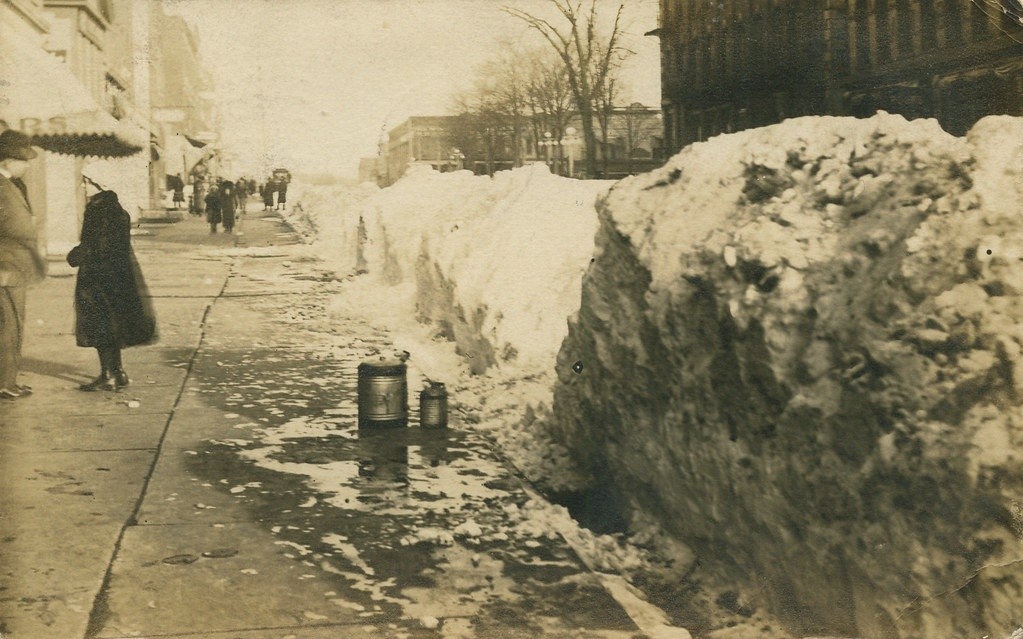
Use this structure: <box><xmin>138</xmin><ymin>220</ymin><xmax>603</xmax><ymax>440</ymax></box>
<box><xmin>1</xmin><ymin>64</ymin><xmax>144</xmax><ymax>159</ymax></box>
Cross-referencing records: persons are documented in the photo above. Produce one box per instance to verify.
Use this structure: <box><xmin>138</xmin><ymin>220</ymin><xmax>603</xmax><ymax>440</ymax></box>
<box><xmin>0</xmin><ymin>128</ymin><xmax>50</xmax><ymax>399</ymax></box>
<box><xmin>172</xmin><ymin>168</ymin><xmax>288</xmax><ymax>232</ymax></box>
<box><xmin>66</xmin><ymin>159</ymin><xmax>160</xmax><ymax>392</ymax></box>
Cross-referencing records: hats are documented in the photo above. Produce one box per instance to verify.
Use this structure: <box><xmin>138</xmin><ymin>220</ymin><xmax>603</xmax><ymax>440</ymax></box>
<box><xmin>82</xmin><ymin>158</ymin><xmax>116</xmax><ymax>194</ymax></box>
<box><xmin>0</xmin><ymin>130</ymin><xmax>38</xmax><ymax>161</ymax></box>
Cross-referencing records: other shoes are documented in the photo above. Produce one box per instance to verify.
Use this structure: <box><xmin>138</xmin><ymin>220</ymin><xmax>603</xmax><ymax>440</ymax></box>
<box><xmin>0</xmin><ymin>386</ymin><xmax>33</xmax><ymax>402</ymax></box>
<box><xmin>79</xmin><ymin>372</ymin><xmax>130</xmax><ymax>393</ymax></box>
